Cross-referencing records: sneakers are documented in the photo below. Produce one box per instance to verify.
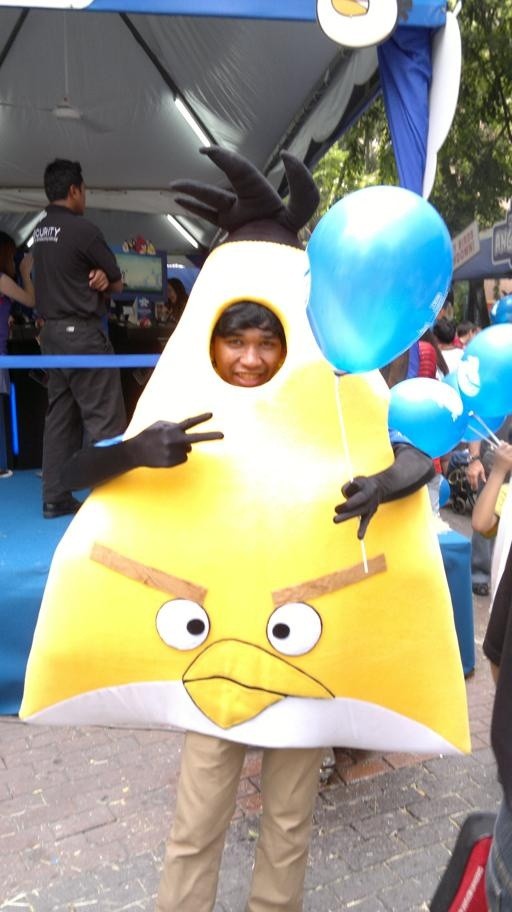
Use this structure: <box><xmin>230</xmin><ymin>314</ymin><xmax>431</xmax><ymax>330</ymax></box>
<box><xmin>42</xmin><ymin>496</ymin><xmax>82</xmax><ymax>519</ymax></box>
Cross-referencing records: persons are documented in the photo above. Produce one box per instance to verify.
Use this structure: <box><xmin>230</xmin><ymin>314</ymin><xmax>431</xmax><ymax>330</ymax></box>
<box><xmin>1</xmin><ymin>161</ymin><xmax>512</xmax><ymax>911</ymax></box>
